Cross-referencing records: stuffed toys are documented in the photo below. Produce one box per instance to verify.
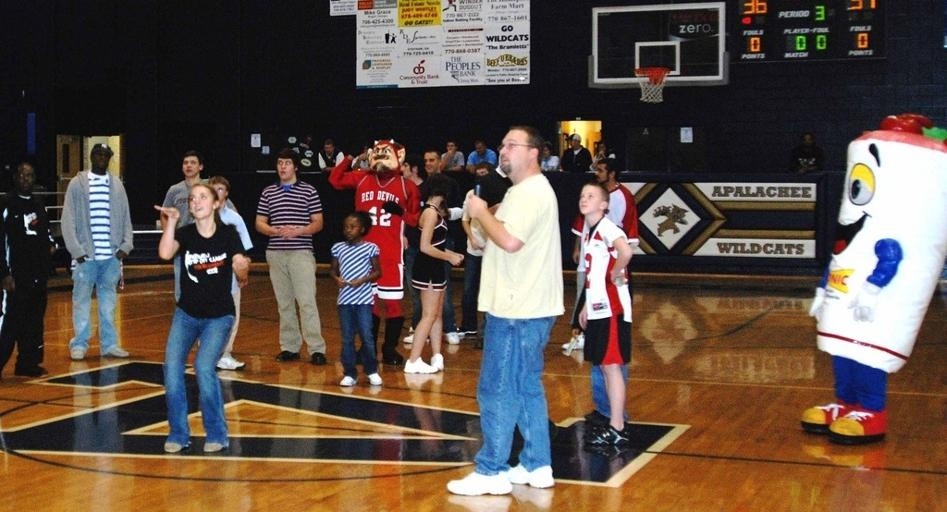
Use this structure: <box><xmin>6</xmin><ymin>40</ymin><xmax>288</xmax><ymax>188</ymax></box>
<box><xmin>799</xmin><ymin>114</ymin><xmax>946</xmax><ymax>446</ymax></box>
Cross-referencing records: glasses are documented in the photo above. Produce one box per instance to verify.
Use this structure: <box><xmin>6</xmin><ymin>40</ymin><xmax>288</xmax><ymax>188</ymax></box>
<box><xmin>498</xmin><ymin>142</ymin><xmax>533</xmax><ymax>151</ymax></box>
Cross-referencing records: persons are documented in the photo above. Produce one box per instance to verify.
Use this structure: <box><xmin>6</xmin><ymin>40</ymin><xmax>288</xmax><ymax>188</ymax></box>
<box><xmin>573</xmin><ymin>182</ymin><xmax>635</xmax><ymax>449</ymax></box>
<box><xmin>170</xmin><ymin>177</ymin><xmax>251</xmax><ymax>372</ymax></box>
<box><xmin>157</xmin><ymin>147</ymin><xmax>239</xmax><ymax>309</ymax></box>
<box><xmin>149</xmin><ymin>182</ymin><xmax>252</xmax><ymax>457</ymax></box>
<box><xmin>560</xmin><ymin>157</ymin><xmax>640</xmax><ymax>352</ymax></box>
<box><xmin>1</xmin><ymin>159</ymin><xmax>59</xmax><ymax>380</ymax></box>
<box><xmin>443</xmin><ymin>123</ymin><xmax>567</xmax><ymax>498</ymax></box>
<box><xmin>570</xmin><ymin>203</ymin><xmax>635</xmax><ymax>427</ymax></box>
<box><xmin>589</xmin><ymin>140</ymin><xmax>617</xmax><ymax>171</ymax></box>
<box><xmin>61</xmin><ymin>144</ymin><xmax>136</xmax><ymax>360</ymax></box>
<box><xmin>559</xmin><ymin>133</ymin><xmax>594</xmax><ymax>171</ymax></box>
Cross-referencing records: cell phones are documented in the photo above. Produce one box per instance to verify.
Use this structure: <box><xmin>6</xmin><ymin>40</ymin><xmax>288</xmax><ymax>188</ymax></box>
<box><xmin>474</xmin><ymin>185</ymin><xmax>480</xmax><ymax>199</ymax></box>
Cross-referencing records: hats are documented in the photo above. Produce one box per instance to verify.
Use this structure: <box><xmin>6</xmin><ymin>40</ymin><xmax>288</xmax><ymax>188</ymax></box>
<box><xmin>566</xmin><ymin>134</ymin><xmax>581</xmax><ymax>141</ymax></box>
<box><xmin>92</xmin><ymin>144</ymin><xmax>114</xmax><ymax>156</ymax></box>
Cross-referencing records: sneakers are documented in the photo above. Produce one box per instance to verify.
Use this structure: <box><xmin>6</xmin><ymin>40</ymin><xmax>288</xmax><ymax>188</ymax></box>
<box><xmin>405</xmin><ymin>353</ymin><xmax>444</xmax><ymax>374</ymax></box>
<box><xmin>202</xmin><ymin>442</ymin><xmax>223</xmax><ymax>452</ymax></box>
<box><xmin>577</xmin><ymin>410</ymin><xmax>630</xmax><ymax>453</ymax></box>
<box><xmin>14</xmin><ymin>360</ymin><xmax>48</xmax><ymax>378</ymax></box>
<box><xmin>68</xmin><ymin>345</ymin><xmax>88</xmax><ymax>360</ymax></box>
<box><xmin>165</xmin><ymin>442</ymin><xmax>183</xmax><ymax>453</ymax></box>
<box><xmin>403</xmin><ymin>327</ymin><xmax>588</xmax><ymax>351</ymax></box>
<box><xmin>366</xmin><ymin>372</ymin><xmax>383</xmax><ymax>385</ymax></box>
<box><xmin>340</xmin><ymin>375</ymin><xmax>359</xmax><ymax>388</ymax></box>
<box><xmin>276</xmin><ymin>350</ymin><xmax>300</xmax><ymax>362</ymax></box>
<box><xmin>215</xmin><ymin>356</ymin><xmax>244</xmax><ymax>372</ymax></box>
<box><xmin>356</xmin><ymin>347</ymin><xmax>402</xmax><ymax>365</ymax></box>
<box><xmin>447</xmin><ymin>460</ymin><xmax>558</xmax><ymax>497</ymax></box>
<box><xmin>105</xmin><ymin>346</ymin><xmax>130</xmax><ymax>359</ymax></box>
<box><xmin>310</xmin><ymin>352</ymin><xmax>327</xmax><ymax>364</ymax></box>
